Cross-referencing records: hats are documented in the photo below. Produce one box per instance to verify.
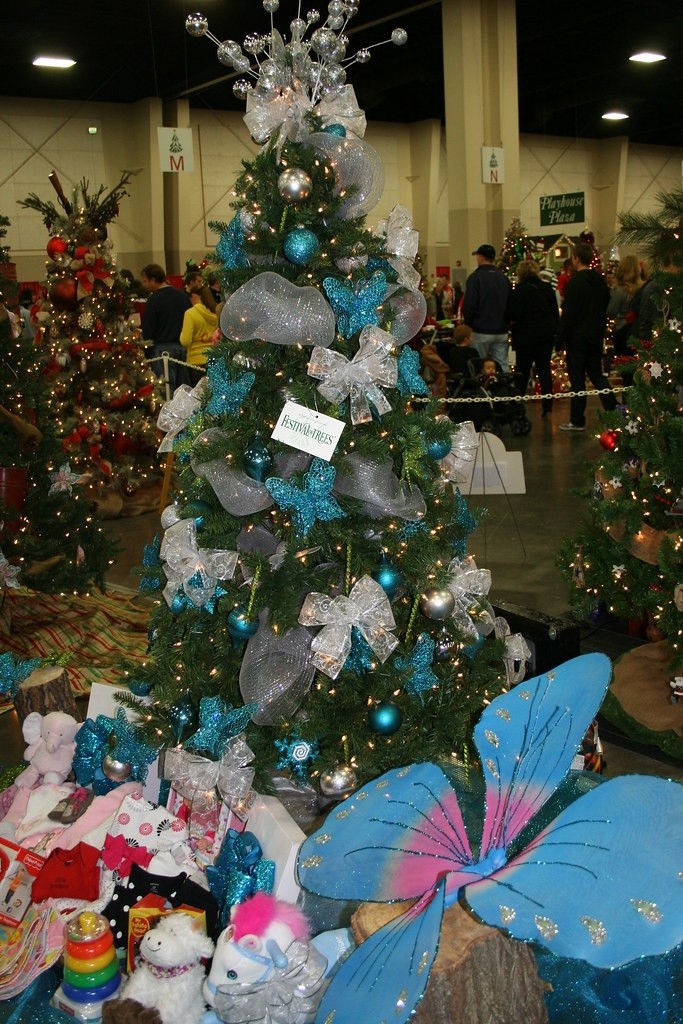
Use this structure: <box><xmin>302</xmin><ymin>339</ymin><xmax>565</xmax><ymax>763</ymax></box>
<box><xmin>471</xmin><ymin>243</ymin><xmax>496</xmax><ymax>260</ymax></box>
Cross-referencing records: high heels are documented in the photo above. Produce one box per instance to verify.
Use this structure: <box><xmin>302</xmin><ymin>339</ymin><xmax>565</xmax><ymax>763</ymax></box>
<box><xmin>539</xmin><ymin>407</ymin><xmax>553</xmax><ymax>420</ymax></box>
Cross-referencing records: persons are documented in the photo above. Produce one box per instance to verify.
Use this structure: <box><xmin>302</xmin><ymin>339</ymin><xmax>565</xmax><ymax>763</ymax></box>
<box><xmin>557</xmin><ymin>239</ymin><xmax>623</xmax><ymax>431</ymax></box>
<box><xmin>184</xmin><ymin>272</ymin><xmax>204</xmax><ymax>307</ymax></box>
<box><xmin>463</xmin><ymin>242</ymin><xmax>516</xmax><ymax>378</ymax></box>
<box><xmin>207</xmin><ymin>269</ymin><xmax>224</xmax><ymax>306</ymax></box>
<box><xmin>418</xmin><ymin>255</ymin><xmax>683</xmax><ymax>433</ymax></box>
<box><xmin>179</xmin><ymin>284</ymin><xmax>220</xmax><ymax>389</ymax></box>
<box><xmin>0</xmin><ymin>271</ymin><xmax>55</xmax><ymax>399</ymax></box>
<box><xmin>117</xmin><ymin>267</ymin><xmax>136</xmax><ymax>304</ymax></box>
<box><xmin>124</xmin><ymin>279</ymin><xmax>148</xmax><ymax>307</ymax></box>
<box><xmin>140</xmin><ymin>262</ymin><xmax>193</xmax><ymax>404</ymax></box>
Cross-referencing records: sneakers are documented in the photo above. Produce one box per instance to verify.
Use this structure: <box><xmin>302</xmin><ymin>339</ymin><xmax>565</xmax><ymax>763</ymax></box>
<box><xmin>560</xmin><ymin>422</ymin><xmax>588</xmax><ymax>431</ymax></box>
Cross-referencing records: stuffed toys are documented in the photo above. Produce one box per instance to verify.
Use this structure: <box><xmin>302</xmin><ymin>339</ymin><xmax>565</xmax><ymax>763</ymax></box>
<box><xmin>99</xmin><ymin>912</ymin><xmax>216</xmax><ymax>1024</ymax></box>
<box><xmin>202</xmin><ymin>894</ymin><xmax>337</xmax><ymax>1024</ymax></box>
<box><xmin>14</xmin><ymin>710</ymin><xmax>88</xmax><ymax>791</ymax></box>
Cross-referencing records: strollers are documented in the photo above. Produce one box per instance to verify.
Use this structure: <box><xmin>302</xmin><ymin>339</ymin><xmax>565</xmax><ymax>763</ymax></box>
<box><xmin>438</xmin><ymin>346</ymin><xmax>534</xmax><ymax>438</ymax></box>
